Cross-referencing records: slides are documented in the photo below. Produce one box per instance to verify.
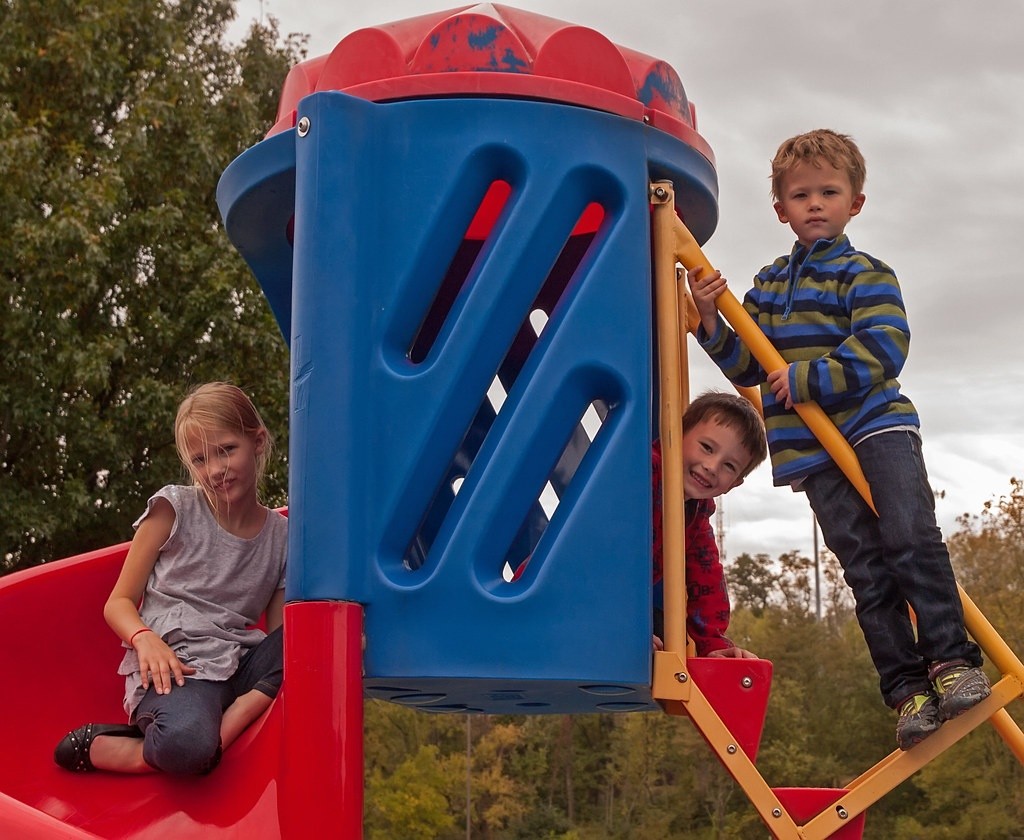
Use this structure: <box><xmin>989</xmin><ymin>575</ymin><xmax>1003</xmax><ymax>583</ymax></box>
<box><xmin>1</xmin><ymin>504</ymin><xmax>362</xmax><ymax>840</ymax></box>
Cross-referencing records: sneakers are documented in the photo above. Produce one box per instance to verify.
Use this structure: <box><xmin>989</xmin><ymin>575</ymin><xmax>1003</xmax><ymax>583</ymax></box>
<box><xmin>896</xmin><ymin>682</ymin><xmax>942</xmax><ymax>752</ymax></box>
<box><xmin>929</xmin><ymin>658</ymin><xmax>993</xmax><ymax>722</ymax></box>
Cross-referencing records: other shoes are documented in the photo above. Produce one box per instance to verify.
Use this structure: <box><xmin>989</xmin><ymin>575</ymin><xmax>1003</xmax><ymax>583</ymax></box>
<box><xmin>54</xmin><ymin>722</ymin><xmax>146</xmax><ymax>773</ymax></box>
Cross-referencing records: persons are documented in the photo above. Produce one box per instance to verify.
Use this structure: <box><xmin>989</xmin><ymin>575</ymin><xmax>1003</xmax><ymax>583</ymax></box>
<box><xmin>686</xmin><ymin>128</ymin><xmax>991</xmax><ymax>752</ymax></box>
<box><xmin>54</xmin><ymin>381</ymin><xmax>289</xmax><ymax>776</ymax></box>
<box><xmin>509</xmin><ymin>388</ymin><xmax>768</xmax><ymax>660</ymax></box>
